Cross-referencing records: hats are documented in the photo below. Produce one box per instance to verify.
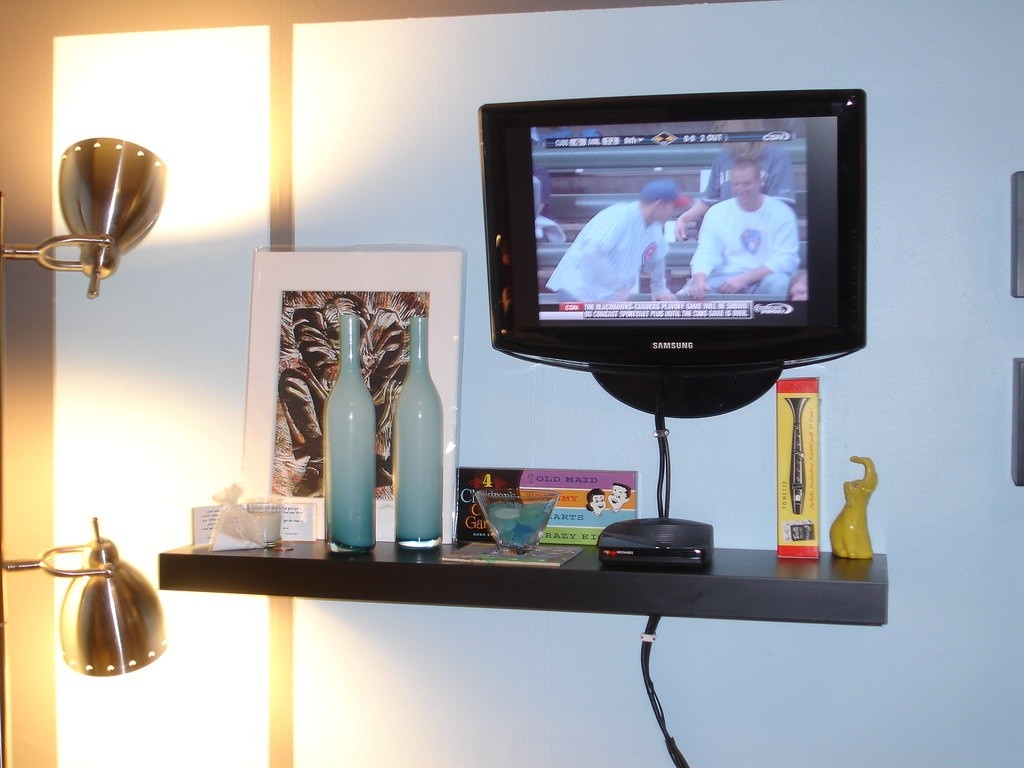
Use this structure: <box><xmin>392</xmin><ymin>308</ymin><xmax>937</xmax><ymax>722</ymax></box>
<box><xmin>642</xmin><ymin>178</ymin><xmax>692</xmax><ymax>207</ymax></box>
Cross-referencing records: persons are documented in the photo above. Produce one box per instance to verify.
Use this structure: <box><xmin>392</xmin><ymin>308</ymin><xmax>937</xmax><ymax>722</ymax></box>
<box><xmin>531</xmin><ymin>120</ymin><xmax>809</xmax><ymax>301</ymax></box>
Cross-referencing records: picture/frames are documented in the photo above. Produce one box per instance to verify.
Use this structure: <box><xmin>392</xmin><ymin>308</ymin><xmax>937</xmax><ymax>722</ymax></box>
<box><xmin>237</xmin><ymin>246</ymin><xmax>466</xmax><ymax>547</ymax></box>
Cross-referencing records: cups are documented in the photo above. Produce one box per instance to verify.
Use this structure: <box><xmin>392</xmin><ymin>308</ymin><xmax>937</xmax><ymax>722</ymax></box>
<box><xmin>247</xmin><ymin>497</ymin><xmax>283</xmax><ymax>547</ymax></box>
<box><xmin>475</xmin><ymin>489</ymin><xmax>561</xmax><ymax>557</ymax></box>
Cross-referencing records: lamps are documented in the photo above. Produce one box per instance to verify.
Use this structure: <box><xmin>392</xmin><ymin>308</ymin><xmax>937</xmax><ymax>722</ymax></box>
<box><xmin>0</xmin><ymin>514</ymin><xmax>169</xmax><ymax>677</ymax></box>
<box><xmin>0</xmin><ymin>136</ymin><xmax>170</xmax><ymax>301</ymax></box>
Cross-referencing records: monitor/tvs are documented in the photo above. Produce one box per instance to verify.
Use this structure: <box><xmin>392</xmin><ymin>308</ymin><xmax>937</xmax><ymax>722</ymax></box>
<box><xmin>478</xmin><ymin>87</ymin><xmax>869</xmax><ymax>377</ymax></box>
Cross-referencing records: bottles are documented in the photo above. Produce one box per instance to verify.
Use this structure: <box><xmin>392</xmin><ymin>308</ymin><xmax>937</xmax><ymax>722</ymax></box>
<box><xmin>393</xmin><ymin>316</ymin><xmax>444</xmax><ymax>553</ymax></box>
<box><xmin>323</xmin><ymin>313</ymin><xmax>376</xmax><ymax>557</ymax></box>
